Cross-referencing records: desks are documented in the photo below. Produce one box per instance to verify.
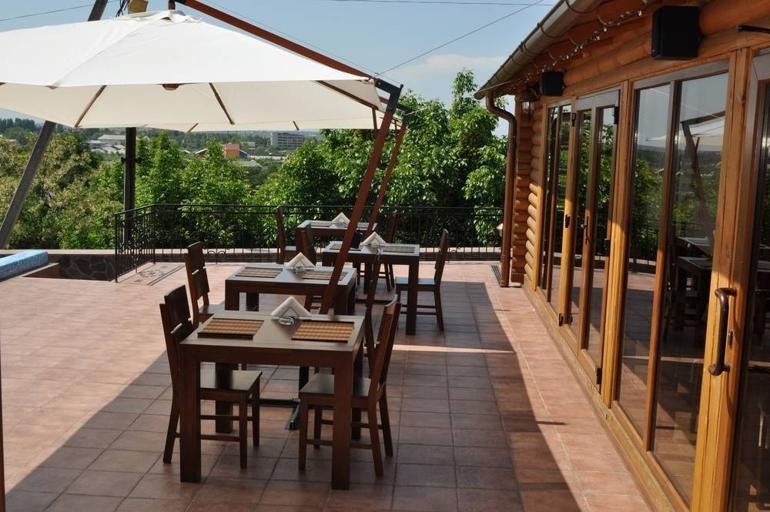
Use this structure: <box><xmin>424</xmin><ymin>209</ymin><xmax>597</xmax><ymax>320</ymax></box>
<box><xmin>296</xmin><ymin>218</ymin><xmax>375</xmax><ymax>286</ymax></box>
<box><xmin>322</xmin><ymin>239</ymin><xmax>419</xmax><ymax>337</ymax></box>
<box><xmin>225</xmin><ymin>263</ymin><xmax>357</xmax><ymax>370</ymax></box>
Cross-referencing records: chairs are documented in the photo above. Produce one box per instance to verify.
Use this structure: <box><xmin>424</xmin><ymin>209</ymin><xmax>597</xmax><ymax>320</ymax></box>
<box><xmin>294</xmin><ymin>220</ymin><xmax>358</xmax><ymax>318</ymax></box>
<box><xmin>161</xmin><ymin>286</ymin><xmax>264</xmax><ymax>472</ymax></box>
<box><xmin>184</xmin><ymin>243</ymin><xmax>263</xmax><ymax>404</ymax></box>
<box><xmin>391</xmin><ymin>229</ymin><xmax>450</xmax><ymax>331</ymax></box>
<box><xmin>273</xmin><ymin>207</ymin><xmax>307</xmax><ymax>263</ymax></box>
<box><xmin>295</xmin><ymin>294</ymin><xmax>401</xmax><ymax>475</ymax></box>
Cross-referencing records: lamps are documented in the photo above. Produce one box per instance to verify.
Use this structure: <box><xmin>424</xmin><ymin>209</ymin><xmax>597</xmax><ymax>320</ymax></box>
<box><xmin>518</xmin><ymin>81</ymin><xmax>541</xmax><ymax>122</ymax></box>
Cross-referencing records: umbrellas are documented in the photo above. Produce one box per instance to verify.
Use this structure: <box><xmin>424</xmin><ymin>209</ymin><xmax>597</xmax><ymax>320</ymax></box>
<box><xmin>140</xmin><ymin>94</ymin><xmax>416</xmax><ymax>241</ymax></box>
<box><xmin>0</xmin><ymin>0</ymin><xmax>406</xmax><ymax>315</ymax></box>
